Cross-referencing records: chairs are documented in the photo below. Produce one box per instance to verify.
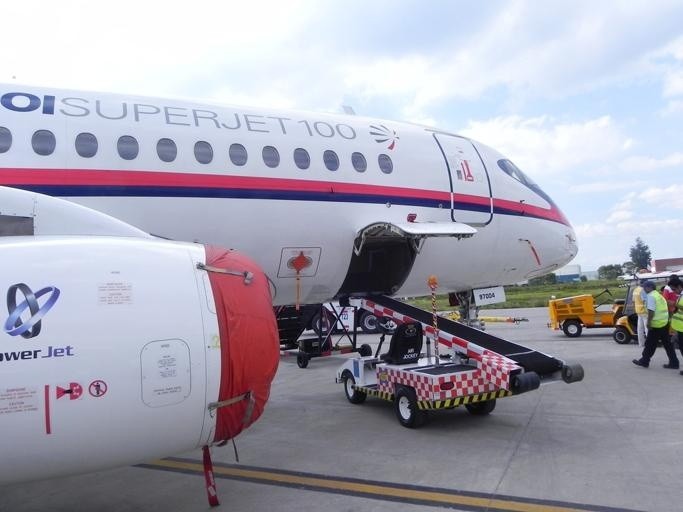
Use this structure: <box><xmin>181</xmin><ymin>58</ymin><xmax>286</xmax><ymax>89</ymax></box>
<box><xmin>381</xmin><ymin>321</ymin><xmax>422</xmax><ymax>364</ymax></box>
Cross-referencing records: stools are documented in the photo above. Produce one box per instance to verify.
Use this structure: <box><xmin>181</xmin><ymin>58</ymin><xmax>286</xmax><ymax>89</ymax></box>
<box><xmin>614</xmin><ymin>299</ymin><xmax>625</xmax><ymax>305</ymax></box>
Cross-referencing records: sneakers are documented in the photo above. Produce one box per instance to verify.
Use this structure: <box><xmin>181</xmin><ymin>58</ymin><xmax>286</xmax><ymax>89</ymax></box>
<box><xmin>663</xmin><ymin>363</ymin><xmax>679</xmax><ymax>368</ymax></box>
<box><xmin>632</xmin><ymin>359</ymin><xmax>649</xmax><ymax>367</ymax></box>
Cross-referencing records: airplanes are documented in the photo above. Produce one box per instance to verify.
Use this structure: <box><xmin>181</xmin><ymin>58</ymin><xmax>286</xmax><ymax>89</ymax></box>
<box><xmin>0</xmin><ymin>86</ymin><xmax>579</xmax><ymax>488</ymax></box>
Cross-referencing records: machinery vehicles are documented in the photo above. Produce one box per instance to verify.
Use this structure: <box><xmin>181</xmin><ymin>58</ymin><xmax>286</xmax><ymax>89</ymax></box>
<box><xmin>311</xmin><ymin>295</ymin><xmax>380</xmax><ymax>337</ymax></box>
<box><xmin>547</xmin><ymin>269</ymin><xmax>683</xmax><ymax>349</ymax></box>
<box><xmin>335</xmin><ymin>274</ymin><xmax>585</xmax><ymax>428</ymax></box>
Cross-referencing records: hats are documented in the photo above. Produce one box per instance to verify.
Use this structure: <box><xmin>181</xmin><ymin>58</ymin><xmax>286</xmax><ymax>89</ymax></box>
<box><xmin>639</xmin><ymin>281</ymin><xmax>656</xmax><ymax>290</ymax></box>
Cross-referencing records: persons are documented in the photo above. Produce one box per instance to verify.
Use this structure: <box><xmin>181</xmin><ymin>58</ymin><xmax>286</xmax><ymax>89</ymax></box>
<box><xmin>670</xmin><ymin>279</ymin><xmax>683</xmax><ymax>376</ymax></box>
<box><xmin>663</xmin><ymin>275</ymin><xmax>679</xmax><ymax>316</ymax></box>
<box><xmin>632</xmin><ymin>279</ymin><xmax>649</xmax><ymax>346</ymax></box>
<box><xmin>632</xmin><ymin>281</ymin><xmax>679</xmax><ymax>368</ymax></box>
